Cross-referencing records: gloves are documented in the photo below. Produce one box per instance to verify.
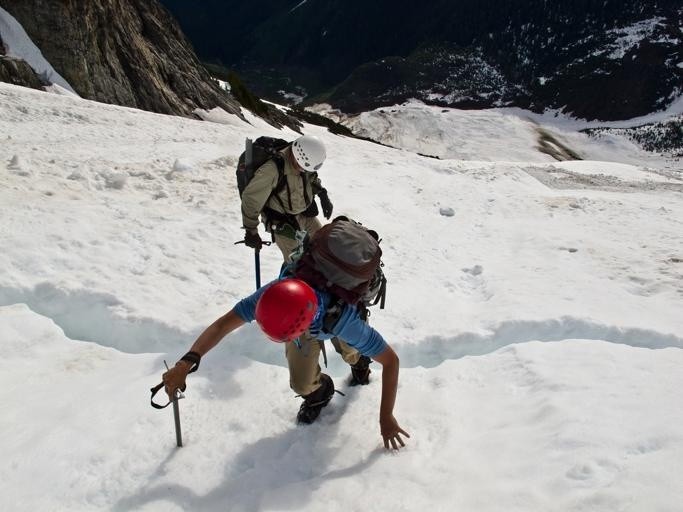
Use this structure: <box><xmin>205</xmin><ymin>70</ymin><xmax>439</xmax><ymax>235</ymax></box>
<box><xmin>318</xmin><ymin>188</ymin><xmax>333</xmax><ymax>220</ymax></box>
<box><xmin>243</xmin><ymin>232</ymin><xmax>261</xmax><ymax>250</ymax></box>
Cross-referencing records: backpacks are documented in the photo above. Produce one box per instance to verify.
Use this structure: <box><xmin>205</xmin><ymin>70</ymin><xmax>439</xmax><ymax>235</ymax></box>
<box><xmin>236</xmin><ymin>135</ymin><xmax>288</xmax><ymax>196</ymax></box>
<box><xmin>285</xmin><ymin>214</ymin><xmax>387</xmax><ymax>334</ymax></box>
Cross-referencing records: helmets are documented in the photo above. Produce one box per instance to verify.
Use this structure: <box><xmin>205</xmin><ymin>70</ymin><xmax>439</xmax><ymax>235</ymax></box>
<box><xmin>255</xmin><ymin>278</ymin><xmax>318</xmax><ymax>343</ymax></box>
<box><xmin>291</xmin><ymin>135</ymin><xmax>327</xmax><ymax>173</ymax></box>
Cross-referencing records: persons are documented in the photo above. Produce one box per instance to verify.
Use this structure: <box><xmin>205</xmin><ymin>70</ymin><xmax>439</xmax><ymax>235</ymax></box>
<box><xmin>240</xmin><ymin>134</ymin><xmax>333</xmax><ymax>273</ymax></box>
<box><xmin>161</xmin><ymin>244</ymin><xmax>410</xmax><ymax>451</ymax></box>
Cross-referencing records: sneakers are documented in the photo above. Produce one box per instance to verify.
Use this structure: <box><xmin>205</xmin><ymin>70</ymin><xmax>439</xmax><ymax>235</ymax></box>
<box><xmin>295</xmin><ymin>373</ymin><xmax>345</xmax><ymax>424</ymax></box>
<box><xmin>348</xmin><ymin>356</ymin><xmax>373</xmax><ymax>387</ymax></box>
